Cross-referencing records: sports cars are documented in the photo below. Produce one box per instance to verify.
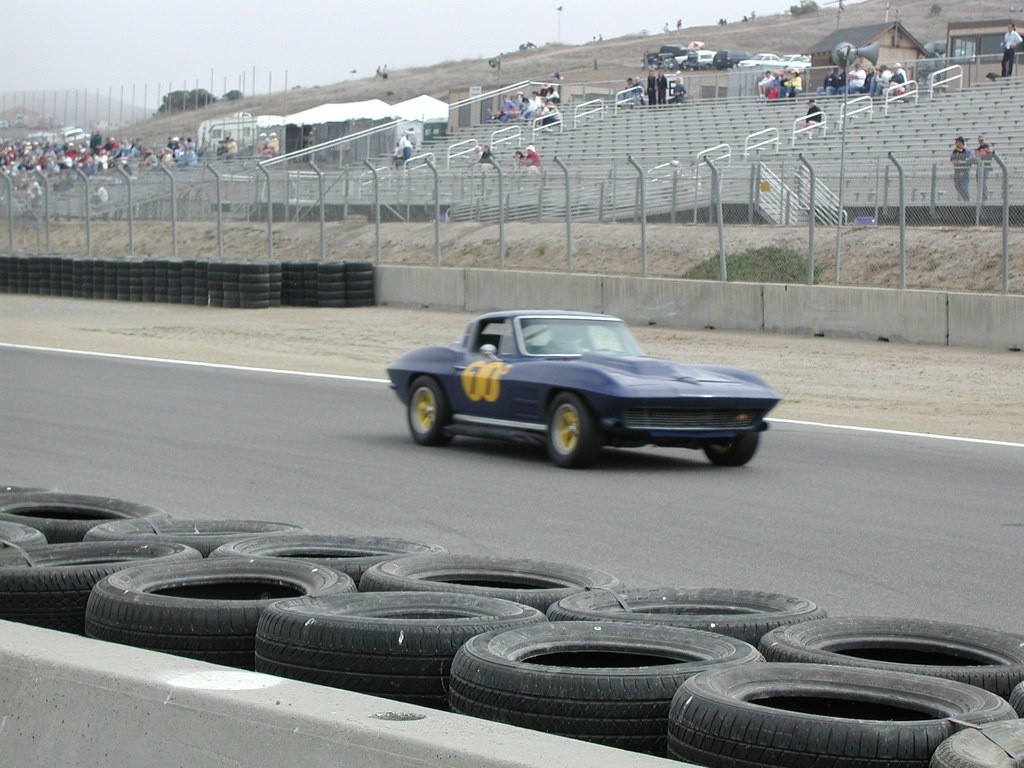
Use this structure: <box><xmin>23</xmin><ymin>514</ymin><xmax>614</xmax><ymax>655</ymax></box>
<box><xmin>385</xmin><ymin>307</ymin><xmax>783</xmax><ymax>468</ymax></box>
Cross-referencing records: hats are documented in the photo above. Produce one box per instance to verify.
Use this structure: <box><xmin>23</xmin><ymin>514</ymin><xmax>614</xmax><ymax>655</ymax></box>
<box><xmin>954</xmin><ymin>137</ymin><xmax>964</xmax><ymax>141</ymax></box>
<box><xmin>516</xmin><ymin>91</ymin><xmax>523</xmax><ymax>96</ymax></box>
<box><xmin>893</xmin><ymin>63</ymin><xmax>900</xmax><ymax>69</ymax></box>
<box><xmin>541</xmin><ymin>82</ymin><xmax>550</xmax><ymax>90</ymax></box>
<box><xmin>791</xmin><ymin>69</ymin><xmax>800</xmax><ymax>75</ymax></box>
<box><xmin>626</xmin><ymin>78</ymin><xmax>632</xmax><ymax>82</ymax></box>
<box><xmin>526</xmin><ymin>145</ymin><xmax>536</xmax><ymax>154</ymax></box>
<box><xmin>1</xmin><ymin>133</ymin><xmax>277</xmax><ymax>173</ymax></box>
<box><xmin>807</xmin><ymin>99</ymin><xmax>814</xmax><ymax>103</ymax></box>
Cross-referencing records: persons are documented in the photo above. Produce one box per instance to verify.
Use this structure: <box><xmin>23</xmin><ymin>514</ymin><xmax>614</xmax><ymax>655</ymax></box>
<box><xmin>303</xmin><ymin>129</ymin><xmax>316</xmax><ymax>163</ymax></box>
<box><xmin>656</xmin><ymin>71</ymin><xmax>667</xmax><ymax>108</ymax></box>
<box><xmin>377</xmin><ymin>62</ymin><xmax>388</xmax><ymax>79</ymax></box>
<box><xmin>794</xmin><ymin>99</ymin><xmax>822</xmax><ymax>135</ymax></box>
<box><xmin>647</xmin><ymin>69</ymin><xmax>657</xmax><ymax>112</ymax></box>
<box><xmin>470</xmin><ymin>142</ymin><xmax>541</xmax><ymax>178</ymax></box>
<box><xmin>1000</xmin><ymin>22</ymin><xmax>1023</xmax><ymax>76</ymax></box>
<box><xmin>668</xmin><ymin>78</ymin><xmax>687</xmax><ymax>104</ymax></box>
<box><xmin>0</xmin><ymin>123</ymin><xmax>280</xmax><ymax>210</ymax></box>
<box><xmin>623</xmin><ymin>78</ymin><xmax>647</xmax><ymax>113</ymax></box>
<box><xmin>951</xmin><ymin>135</ymin><xmax>973</xmax><ymax>204</ymax></box>
<box><xmin>391</xmin><ymin>133</ymin><xmax>415</xmax><ymax>171</ymax></box>
<box><xmin>494</xmin><ymin>83</ymin><xmax>562</xmax><ymax>132</ymax></box>
<box><xmin>973</xmin><ymin>134</ymin><xmax>994</xmax><ymax>203</ymax></box>
<box><xmin>757</xmin><ymin>62</ymin><xmax>909</xmax><ymax>106</ymax></box>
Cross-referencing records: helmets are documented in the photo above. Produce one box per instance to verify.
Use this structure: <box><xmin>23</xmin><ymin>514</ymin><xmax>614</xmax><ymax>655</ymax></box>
<box><xmin>551</xmin><ymin>323</ymin><xmax>583</xmax><ymax>353</ymax></box>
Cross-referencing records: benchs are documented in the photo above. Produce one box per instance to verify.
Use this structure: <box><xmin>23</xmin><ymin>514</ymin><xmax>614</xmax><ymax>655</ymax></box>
<box><xmin>0</xmin><ymin>75</ymin><xmax>1024</xmax><ymax>219</ymax></box>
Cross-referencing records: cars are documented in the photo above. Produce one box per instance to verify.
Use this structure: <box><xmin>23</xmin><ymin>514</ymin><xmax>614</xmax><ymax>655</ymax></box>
<box><xmin>64</xmin><ymin>128</ymin><xmax>91</xmax><ymax>143</ymax></box>
<box><xmin>772</xmin><ymin>54</ymin><xmax>812</xmax><ymax>73</ymax></box>
<box><xmin>737</xmin><ymin>53</ymin><xmax>780</xmax><ymax>67</ymax></box>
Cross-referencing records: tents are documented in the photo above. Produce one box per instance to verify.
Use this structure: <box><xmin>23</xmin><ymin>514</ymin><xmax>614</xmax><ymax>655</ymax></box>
<box><xmin>249</xmin><ymin>93</ymin><xmax>451</xmax><ymax>150</ymax></box>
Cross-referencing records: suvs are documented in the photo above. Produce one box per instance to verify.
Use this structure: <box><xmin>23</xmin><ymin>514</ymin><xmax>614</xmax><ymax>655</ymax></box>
<box><xmin>713</xmin><ymin>49</ymin><xmax>753</xmax><ymax>71</ymax></box>
<box><xmin>640</xmin><ymin>44</ymin><xmax>716</xmax><ymax>72</ymax></box>
